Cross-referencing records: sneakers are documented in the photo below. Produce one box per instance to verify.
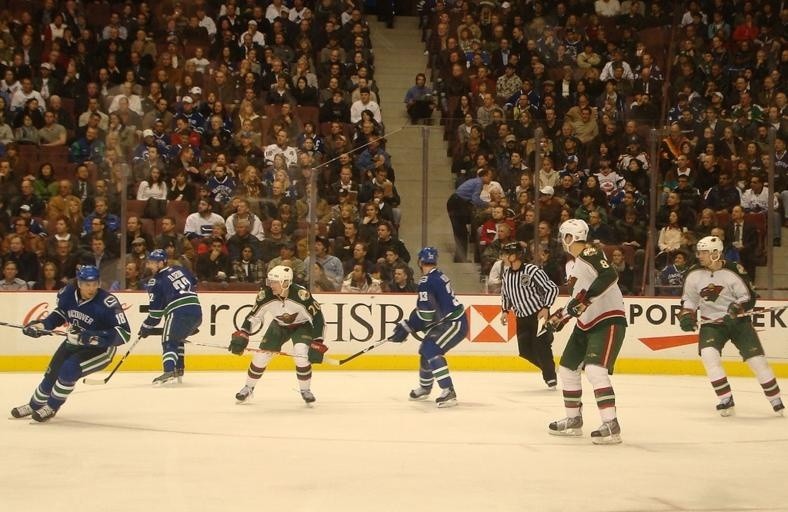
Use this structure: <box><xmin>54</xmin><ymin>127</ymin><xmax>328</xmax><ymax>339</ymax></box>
<box><xmin>435</xmin><ymin>385</ymin><xmax>456</xmax><ymax>402</ymax></box>
<box><xmin>770</xmin><ymin>396</ymin><xmax>785</xmax><ymax>412</ymax></box>
<box><xmin>409</xmin><ymin>386</ymin><xmax>431</xmax><ymax>398</ymax></box>
<box><xmin>11</xmin><ymin>404</ymin><xmax>34</xmax><ymax>418</ymax></box>
<box><xmin>300</xmin><ymin>389</ymin><xmax>316</xmax><ymax>402</ymax></box>
<box><xmin>153</xmin><ymin>370</ymin><xmax>175</xmax><ymax>382</ymax></box>
<box><xmin>545</xmin><ymin>373</ymin><xmax>557</xmax><ymax>387</ymax></box>
<box><xmin>235</xmin><ymin>384</ymin><xmax>254</xmax><ymax>401</ymax></box>
<box><xmin>176</xmin><ymin>368</ymin><xmax>184</xmax><ymax>376</ymax></box>
<box><xmin>549</xmin><ymin>414</ymin><xmax>583</xmax><ymax>431</ymax></box>
<box><xmin>716</xmin><ymin>395</ymin><xmax>734</xmax><ymax>410</ymax></box>
<box><xmin>591</xmin><ymin>419</ymin><xmax>620</xmax><ymax>437</ymax></box>
<box><xmin>32</xmin><ymin>404</ymin><xmax>56</xmax><ymax>422</ymax></box>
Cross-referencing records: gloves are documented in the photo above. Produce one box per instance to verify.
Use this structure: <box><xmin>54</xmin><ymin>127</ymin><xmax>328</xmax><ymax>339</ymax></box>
<box><xmin>542</xmin><ymin>308</ymin><xmax>570</xmax><ymax>333</ymax></box>
<box><xmin>566</xmin><ymin>289</ymin><xmax>592</xmax><ymax>318</ymax></box>
<box><xmin>66</xmin><ymin>325</ymin><xmax>83</xmax><ymax>347</ymax></box>
<box><xmin>308</xmin><ymin>340</ymin><xmax>328</xmax><ymax>364</ymax></box>
<box><xmin>227</xmin><ymin>331</ymin><xmax>249</xmax><ymax>356</ymax></box>
<box><xmin>137</xmin><ymin>321</ymin><xmax>153</xmax><ymax>339</ymax></box>
<box><xmin>22</xmin><ymin>321</ymin><xmax>45</xmax><ymax>338</ymax></box>
<box><xmin>677</xmin><ymin>308</ymin><xmax>698</xmax><ymax>332</ymax></box>
<box><xmin>722</xmin><ymin>301</ymin><xmax>743</xmax><ymax>323</ymax></box>
<box><xmin>387</xmin><ymin>319</ymin><xmax>412</xmax><ymax>343</ymax></box>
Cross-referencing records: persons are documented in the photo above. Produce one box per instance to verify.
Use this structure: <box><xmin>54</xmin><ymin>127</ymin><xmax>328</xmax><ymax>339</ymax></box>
<box><xmin>544</xmin><ymin>218</ymin><xmax>627</xmax><ymax>438</ymax></box>
<box><xmin>0</xmin><ymin>1</ymin><xmax>410</xmax><ymax>293</ymax></box>
<box><xmin>228</xmin><ymin>265</ymin><xmax>326</xmax><ymax>403</ymax></box>
<box><xmin>677</xmin><ymin>236</ymin><xmax>784</xmax><ymax>412</ymax></box>
<box><xmin>11</xmin><ymin>264</ymin><xmax>131</xmax><ymax>422</ymax></box>
<box><xmin>419</xmin><ymin>0</ymin><xmax>787</xmax><ymax>293</ymax></box>
<box><xmin>393</xmin><ymin>247</ymin><xmax>468</xmax><ymax>402</ymax></box>
<box><xmin>137</xmin><ymin>248</ymin><xmax>202</xmax><ymax>383</ymax></box>
<box><xmin>404</xmin><ymin>72</ymin><xmax>434</xmax><ymax>126</ymax></box>
<box><xmin>499</xmin><ymin>241</ymin><xmax>560</xmax><ymax>387</ymax></box>
<box><xmin>392</xmin><ymin>264</ymin><xmax>419</xmax><ymax>292</ymax></box>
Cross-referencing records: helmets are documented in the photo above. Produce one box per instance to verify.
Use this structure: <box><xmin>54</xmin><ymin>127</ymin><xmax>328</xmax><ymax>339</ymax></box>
<box><xmin>558</xmin><ymin>218</ymin><xmax>589</xmax><ymax>243</ymax></box>
<box><xmin>147</xmin><ymin>248</ymin><xmax>169</xmax><ymax>263</ymax></box>
<box><xmin>76</xmin><ymin>265</ymin><xmax>100</xmax><ymax>282</ymax></box>
<box><xmin>267</xmin><ymin>264</ymin><xmax>294</xmax><ymax>282</ymax></box>
<box><xmin>418</xmin><ymin>245</ymin><xmax>439</xmax><ymax>264</ymax></box>
<box><xmin>695</xmin><ymin>235</ymin><xmax>724</xmax><ymax>252</ymax></box>
<box><xmin>502</xmin><ymin>241</ymin><xmax>525</xmax><ymax>254</ymax></box>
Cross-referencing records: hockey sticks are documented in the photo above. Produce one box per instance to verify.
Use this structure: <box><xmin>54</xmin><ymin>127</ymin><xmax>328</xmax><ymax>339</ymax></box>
<box><xmin>696</xmin><ymin>306</ymin><xmax>786</xmax><ymax>325</ymax></box>
<box><xmin>177</xmin><ymin>338</ymin><xmax>309</xmax><ymax>357</ymax></box>
<box><xmin>83</xmin><ymin>335</ymin><xmax>141</xmax><ymax>385</ymax></box>
<box><xmin>322</xmin><ymin>337</ymin><xmax>390</xmax><ymax>365</ymax></box>
<box><xmin>536</xmin><ymin>304</ymin><xmax>579</xmax><ymax>337</ymax></box>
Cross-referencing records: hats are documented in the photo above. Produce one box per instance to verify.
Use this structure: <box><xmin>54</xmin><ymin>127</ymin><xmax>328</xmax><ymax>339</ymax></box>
<box><xmin>317</xmin><ymin>234</ymin><xmax>329</xmax><ymax>250</ymax></box>
<box><xmin>188</xmin><ymin>86</ymin><xmax>202</xmax><ymax>95</ymax></box>
<box><xmin>540</xmin><ymin>186</ymin><xmax>555</xmax><ymax>195</ymax></box>
<box><xmin>141</xmin><ymin>129</ymin><xmax>154</xmax><ymax>139</ymax></box>
<box><xmin>565</xmin><ymin>154</ymin><xmax>578</xmax><ymax>162</ymax></box>
<box><xmin>20</xmin><ymin>205</ymin><xmax>32</xmax><ymax>214</ymax></box>
<box><xmin>181</xmin><ymin>95</ymin><xmax>194</xmax><ymax>104</ymax></box>
<box><xmin>504</xmin><ymin>134</ymin><xmax>516</xmax><ymax>143</ymax></box>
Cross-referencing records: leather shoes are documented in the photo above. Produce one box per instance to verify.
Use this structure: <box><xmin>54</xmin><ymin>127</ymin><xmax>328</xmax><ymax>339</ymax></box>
<box><xmin>773</xmin><ymin>238</ymin><xmax>780</xmax><ymax>247</ymax></box>
